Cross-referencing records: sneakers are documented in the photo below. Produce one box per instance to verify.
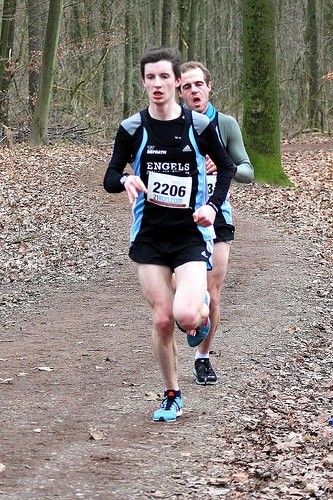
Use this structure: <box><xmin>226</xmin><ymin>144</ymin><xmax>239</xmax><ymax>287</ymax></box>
<box><xmin>193</xmin><ymin>357</ymin><xmax>218</xmax><ymax>385</ymax></box>
<box><xmin>187</xmin><ymin>291</ymin><xmax>212</xmax><ymax>348</ymax></box>
<box><xmin>175</xmin><ymin>321</ymin><xmax>186</xmax><ymax>333</ymax></box>
<box><xmin>152</xmin><ymin>390</ymin><xmax>184</xmax><ymax>421</ymax></box>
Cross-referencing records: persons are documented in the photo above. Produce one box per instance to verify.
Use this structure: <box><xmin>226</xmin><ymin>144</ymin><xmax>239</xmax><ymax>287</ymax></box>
<box><xmin>177</xmin><ymin>61</ymin><xmax>253</xmax><ymax>386</ymax></box>
<box><xmin>104</xmin><ymin>45</ymin><xmax>236</xmax><ymax>427</ymax></box>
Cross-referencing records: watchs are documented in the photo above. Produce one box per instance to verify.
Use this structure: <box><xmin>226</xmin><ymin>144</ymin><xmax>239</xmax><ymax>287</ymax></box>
<box><xmin>120</xmin><ymin>175</ymin><xmax>127</xmax><ymax>190</ymax></box>
